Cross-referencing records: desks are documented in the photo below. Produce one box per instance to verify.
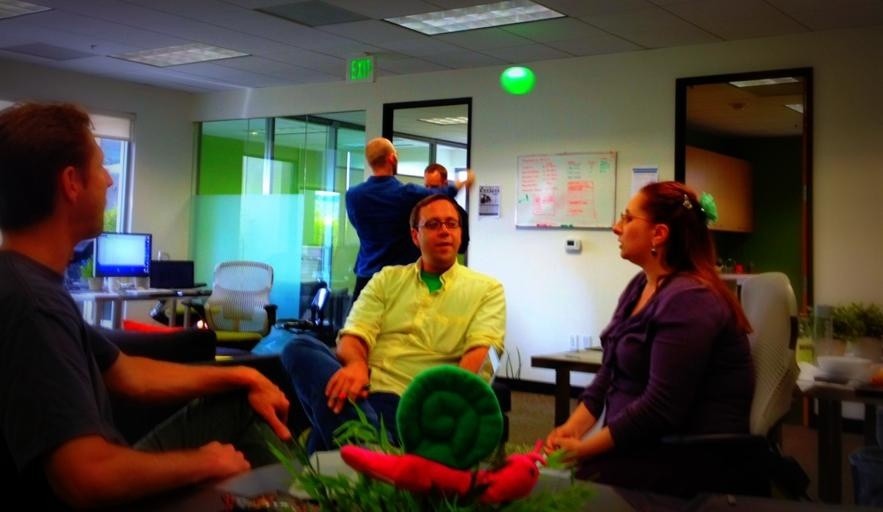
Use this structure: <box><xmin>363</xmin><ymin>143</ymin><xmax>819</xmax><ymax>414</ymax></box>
<box><xmin>532</xmin><ymin>334</ymin><xmax>883</xmax><ymax>504</ymax></box>
<box><xmin>156</xmin><ymin>448</ymin><xmax>853</xmax><ymax>510</ymax></box>
<box><xmin>69</xmin><ymin>287</ymin><xmax>212</xmax><ymax>328</ymax></box>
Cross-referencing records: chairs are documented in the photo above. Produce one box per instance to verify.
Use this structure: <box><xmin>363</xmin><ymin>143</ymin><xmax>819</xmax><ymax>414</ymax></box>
<box><xmin>659</xmin><ymin>271</ymin><xmax>801</xmax><ymax>499</ymax></box>
<box><xmin>182</xmin><ymin>260</ymin><xmax>279</xmax><ymax>351</ymax></box>
<box><xmin>274</xmin><ymin>287</ymin><xmax>331</xmax><ymax>334</ymax></box>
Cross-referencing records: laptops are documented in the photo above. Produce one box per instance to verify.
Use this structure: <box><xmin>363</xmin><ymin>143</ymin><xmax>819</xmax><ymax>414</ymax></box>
<box><xmin>153</xmin><ymin>260</ymin><xmax>206</xmax><ymax>289</ymax></box>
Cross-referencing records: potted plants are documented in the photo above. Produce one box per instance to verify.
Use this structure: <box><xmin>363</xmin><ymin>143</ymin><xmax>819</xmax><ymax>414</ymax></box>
<box><xmin>799</xmin><ymin>300</ymin><xmax>883</xmax><ymax>368</ymax></box>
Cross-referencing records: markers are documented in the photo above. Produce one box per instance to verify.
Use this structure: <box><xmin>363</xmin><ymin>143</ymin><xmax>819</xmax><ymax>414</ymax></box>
<box><xmin>561</xmin><ymin>224</ymin><xmax>574</xmax><ymax>227</ymax></box>
<box><xmin>537</xmin><ymin>224</ymin><xmax>551</xmax><ymax>226</ymax></box>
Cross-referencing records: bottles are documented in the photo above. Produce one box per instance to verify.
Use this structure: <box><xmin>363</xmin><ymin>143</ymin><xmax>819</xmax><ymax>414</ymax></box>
<box><xmin>796</xmin><ymin>311</ymin><xmax>816</xmax><ymax>367</ymax></box>
<box><xmin>715</xmin><ymin>257</ymin><xmax>737</xmax><ymax>275</ymax></box>
<box><xmin>158</xmin><ymin>253</ymin><xmax>168</xmax><ymax>260</ymax></box>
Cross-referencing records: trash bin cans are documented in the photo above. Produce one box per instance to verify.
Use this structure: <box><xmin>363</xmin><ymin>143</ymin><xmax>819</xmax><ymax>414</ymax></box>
<box><xmin>850</xmin><ymin>445</ymin><xmax>882</xmax><ymax>506</ymax></box>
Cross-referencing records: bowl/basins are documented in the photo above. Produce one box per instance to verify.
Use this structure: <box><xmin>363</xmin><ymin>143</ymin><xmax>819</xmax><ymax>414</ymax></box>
<box><xmin>817</xmin><ymin>355</ymin><xmax>871</xmax><ymax>377</ymax></box>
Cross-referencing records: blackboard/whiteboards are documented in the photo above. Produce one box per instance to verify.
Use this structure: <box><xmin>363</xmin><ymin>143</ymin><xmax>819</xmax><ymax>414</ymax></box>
<box><xmin>515</xmin><ymin>152</ymin><xmax>617</xmax><ymax>229</ymax></box>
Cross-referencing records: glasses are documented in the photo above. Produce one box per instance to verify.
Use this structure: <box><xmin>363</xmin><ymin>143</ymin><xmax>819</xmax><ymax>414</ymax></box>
<box><xmin>417</xmin><ymin>219</ymin><xmax>461</xmax><ymax>230</ymax></box>
<box><xmin>620</xmin><ymin>211</ymin><xmax>652</xmax><ymax>225</ymax></box>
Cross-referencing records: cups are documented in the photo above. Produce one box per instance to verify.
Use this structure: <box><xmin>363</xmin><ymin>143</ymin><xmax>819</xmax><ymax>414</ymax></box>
<box><xmin>87</xmin><ymin>276</ymin><xmax>105</xmax><ymax>292</ymax></box>
<box><xmin>109</xmin><ymin>277</ymin><xmax>120</xmax><ymax>292</ymax></box>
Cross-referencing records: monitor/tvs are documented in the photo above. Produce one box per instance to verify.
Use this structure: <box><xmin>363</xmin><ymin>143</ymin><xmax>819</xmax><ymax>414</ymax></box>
<box><xmin>90</xmin><ymin>232</ymin><xmax>152</xmax><ymax>292</ymax></box>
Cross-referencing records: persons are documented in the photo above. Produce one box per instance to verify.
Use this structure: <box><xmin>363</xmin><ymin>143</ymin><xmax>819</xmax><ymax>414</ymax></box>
<box><xmin>424</xmin><ymin>164</ymin><xmax>470</xmax><ymax>257</ymax></box>
<box><xmin>540</xmin><ymin>179</ymin><xmax>758</xmax><ymax>492</ymax></box>
<box><xmin>0</xmin><ymin>101</ymin><xmax>299</xmax><ymax>510</ymax></box>
<box><xmin>280</xmin><ymin>193</ymin><xmax>507</xmax><ymax>460</ymax></box>
<box><xmin>343</xmin><ymin>136</ymin><xmax>473</xmax><ymax>307</ymax></box>
